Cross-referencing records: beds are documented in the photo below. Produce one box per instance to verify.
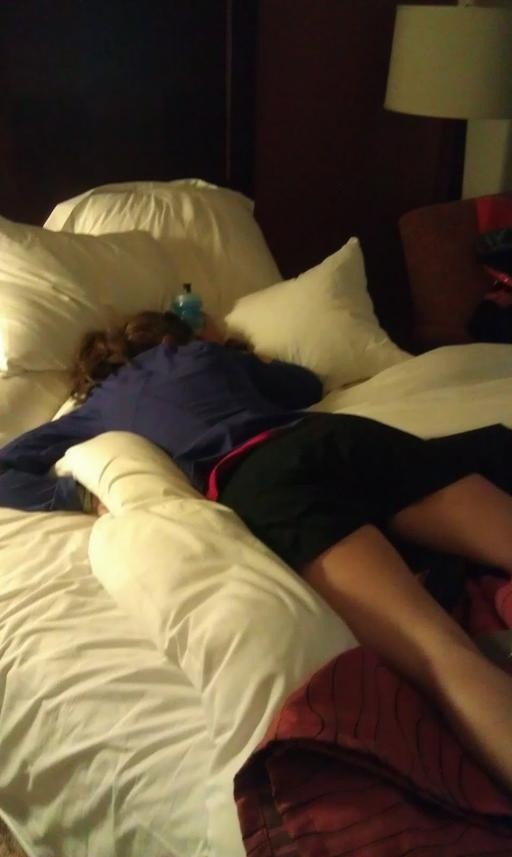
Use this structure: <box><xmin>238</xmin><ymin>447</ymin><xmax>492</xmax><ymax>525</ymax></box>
<box><xmin>1</xmin><ymin>369</ymin><xmax>512</xmax><ymax>857</ymax></box>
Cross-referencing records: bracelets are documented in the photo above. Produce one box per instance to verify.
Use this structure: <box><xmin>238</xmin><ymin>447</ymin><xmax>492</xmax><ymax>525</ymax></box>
<box><xmin>81</xmin><ymin>487</ymin><xmax>96</xmax><ymax>515</ymax></box>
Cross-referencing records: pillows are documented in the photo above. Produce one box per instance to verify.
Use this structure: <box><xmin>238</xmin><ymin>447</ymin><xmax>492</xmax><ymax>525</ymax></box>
<box><xmin>224</xmin><ymin>236</ymin><xmax>417</xmax><ymax>399</ymax></box>
<box><xmin>43</xmin><ymin>177</ymin><xmax>284</xmax><ymax>340</ymax></box>
<box><xmin>0</xmin><ymin>213</ymin><xmax>170</xmax><ymax>376</ymax></box>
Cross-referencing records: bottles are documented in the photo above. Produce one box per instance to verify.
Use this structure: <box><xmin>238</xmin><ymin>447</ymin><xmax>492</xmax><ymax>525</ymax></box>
<box><xmin>172</xmin><ymin>283</ymin><xmax>204</xmax><ymax>337</ymax></box>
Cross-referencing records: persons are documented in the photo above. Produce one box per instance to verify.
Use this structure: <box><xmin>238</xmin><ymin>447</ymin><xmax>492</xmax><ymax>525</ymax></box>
<box><xmin>0</xmin><ymin>309</ymin><xmax>512</xmax><ymax>794</ymax></box>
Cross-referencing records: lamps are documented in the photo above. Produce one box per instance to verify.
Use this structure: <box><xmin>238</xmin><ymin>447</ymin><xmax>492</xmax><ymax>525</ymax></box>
<box><xmin>383</xmin><ymin>0</ymin><xmax>512</xmax><ymax>120</ymax></box>
